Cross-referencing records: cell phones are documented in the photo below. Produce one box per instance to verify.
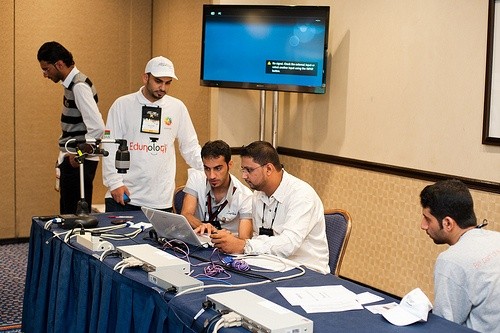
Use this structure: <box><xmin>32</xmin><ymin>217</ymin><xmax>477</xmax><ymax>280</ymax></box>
<box><xmin>39</xmin><ymin>214</ymin><xmax>59</xmax><ymax>220</ymax></box>
<box><xmin>111</xmin><ymin>218</ymin><xmax>132</xmax><ymax>224</ymax></box>
<box><xmin>123</xmin><ymin>193</ymin><xmax>130</xmax><ymax>203</ymax></box>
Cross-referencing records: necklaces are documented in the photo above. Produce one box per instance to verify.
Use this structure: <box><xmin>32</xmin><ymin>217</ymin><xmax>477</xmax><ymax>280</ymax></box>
<box><xmin>455</xmin><ymin>228</ymin><xmax>477</xmax><ymax>241</ymax></box>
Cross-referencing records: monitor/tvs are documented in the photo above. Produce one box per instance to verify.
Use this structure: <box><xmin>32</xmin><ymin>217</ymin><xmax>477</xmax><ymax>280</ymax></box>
<box><xmin>200</xmin><ymin>4</ymin><xmax>331</xmax><ymax>95</ymax></box>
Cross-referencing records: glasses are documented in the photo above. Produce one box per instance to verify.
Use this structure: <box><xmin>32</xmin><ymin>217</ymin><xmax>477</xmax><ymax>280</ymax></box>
<box><xmin>240</xmin><ymin>162</ymin><xmax>273</xmax><ymax>174</ymax></box>
<box><xmin>43</xmin><ymin>62</ymin><xmax>59</xmax><ymax>73</ymax></box>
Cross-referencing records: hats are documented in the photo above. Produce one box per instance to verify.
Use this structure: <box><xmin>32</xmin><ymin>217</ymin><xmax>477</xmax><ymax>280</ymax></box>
<box><xmin>145</xmin><ymin>56</ymin><xmax>178</xmax><ymax>81</ymax></box>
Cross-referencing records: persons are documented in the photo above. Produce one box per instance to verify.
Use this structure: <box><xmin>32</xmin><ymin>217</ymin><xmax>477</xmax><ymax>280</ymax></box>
<box><xmin>420</xmin><ymin>180</ymin><xmax>500</xmax><ymax>333</ymax></box>
<box><xmin>37</xmin><ymin>41</ymin><xmax>105</xmax><ymax>215</ymax></box>
<box><xmin>103</xmin><ymin>55</ymin><xmax>204</xmax><ymax>212</ymax></box>
<box><xmin>182</xmin><ymin>140</ymin><xmax>330</xmax><ymax>274</ymax></box>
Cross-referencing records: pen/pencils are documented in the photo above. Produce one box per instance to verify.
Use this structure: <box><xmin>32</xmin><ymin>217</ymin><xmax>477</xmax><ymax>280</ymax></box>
<box><xmin>106</xmin><ymin>215</ymin><xmax>134</xmax><ymax>219</ymax></box>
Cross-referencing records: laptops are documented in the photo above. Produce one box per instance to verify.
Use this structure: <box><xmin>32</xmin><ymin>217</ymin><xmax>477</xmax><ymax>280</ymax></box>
<box><xmin>140</xmin><ymin>206</ymin><xmax>218</xmax><ymax>248</ymax></box>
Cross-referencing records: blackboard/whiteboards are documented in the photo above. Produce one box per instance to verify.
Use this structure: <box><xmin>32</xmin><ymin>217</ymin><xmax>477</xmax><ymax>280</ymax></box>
<box><xmin>482</xmin><ymin>0</ymin><xmax>500</xmax><ymax>146</ymax></box>
